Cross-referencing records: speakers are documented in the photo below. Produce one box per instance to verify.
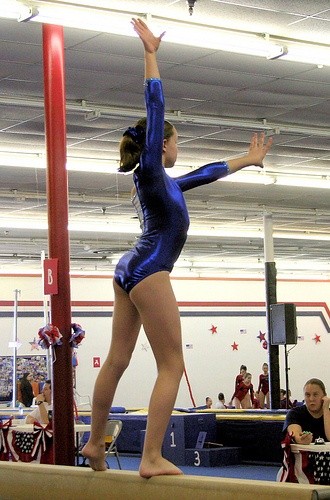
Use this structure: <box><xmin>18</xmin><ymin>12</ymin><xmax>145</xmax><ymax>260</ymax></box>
<box><xmin>270</xmin><ymin>303</ymin><xmax>297</xmax><ymax>345</ymax></box>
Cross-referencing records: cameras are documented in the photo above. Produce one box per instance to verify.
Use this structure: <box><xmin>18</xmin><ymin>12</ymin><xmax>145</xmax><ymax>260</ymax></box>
<box><xmin>315</xmin><ymin>438</ymin><xmax>324</xmax><ymax>443</ymax></box>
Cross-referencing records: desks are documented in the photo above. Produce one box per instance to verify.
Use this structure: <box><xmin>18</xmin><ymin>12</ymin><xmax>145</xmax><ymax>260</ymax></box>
<box><xmin>0</xmin><ymin>402</ymin><xmax>92</xmax><ymax>467</ymax></box>
<box><xmin>291</xmin><ymin>441</ymin><xmax>330</xmax><ymax>453</ymax></box>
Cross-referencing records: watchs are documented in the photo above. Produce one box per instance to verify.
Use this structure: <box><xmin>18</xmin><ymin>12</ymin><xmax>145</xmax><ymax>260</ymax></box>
<box><xmin>37</xmin><ymin>401</ymin><xmax>43</xmax><ymax>405</ymax></box>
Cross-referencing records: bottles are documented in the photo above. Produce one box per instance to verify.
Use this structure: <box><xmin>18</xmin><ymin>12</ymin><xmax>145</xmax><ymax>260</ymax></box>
<box><xmin>19</xmin><ymin>404</ymin><xmax>23</xmax><ymax>415</ymax></box>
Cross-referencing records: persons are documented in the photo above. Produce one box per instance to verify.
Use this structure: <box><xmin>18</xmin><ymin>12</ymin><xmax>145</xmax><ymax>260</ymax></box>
<box><xmin>282</xmin><ymin>378</ymin><xmax>330</xmax><ymax>485</ymax></box>
<box><xmin>206</xmin><ymin>363</ymin><xmax>295</xmax><ymax>409</ymax></box>
<box><xmin>24</xmin><ymin>379</ymin><xmax>51</xmax><ymax>424</ymax></box>
<box><xmin>20</xmin><ymin>373</ymin><xmax>34</xmax><ymax>407</ymax></box>
<box><xmin>81</xmin><ymin>18</ymin><xmax>272</xmax><ymax>478</ymax></box>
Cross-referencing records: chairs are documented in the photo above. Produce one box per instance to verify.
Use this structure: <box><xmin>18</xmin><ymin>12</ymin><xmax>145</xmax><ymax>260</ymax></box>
<box><xmin>75</xmin><ymin>420</ymin><xmax>122</xmax><ymax>470</ymax></box>
